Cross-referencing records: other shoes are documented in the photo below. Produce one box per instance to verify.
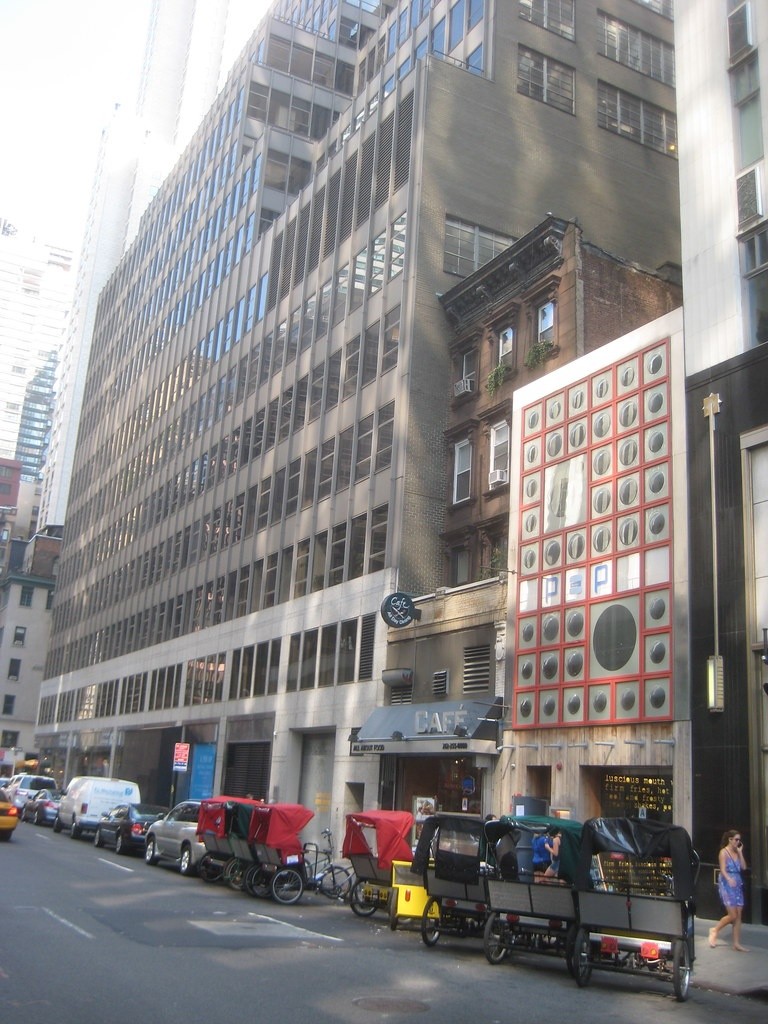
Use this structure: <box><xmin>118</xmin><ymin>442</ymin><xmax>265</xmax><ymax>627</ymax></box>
<box><xmin>732</xmin><ymin>943</ymin><xmax>751</xmax><ymax>952</ymax></box>
<box><xmin>709</xmin><ymin>927</ymin><xmax>716</xmax><ymax>947</ymax></box>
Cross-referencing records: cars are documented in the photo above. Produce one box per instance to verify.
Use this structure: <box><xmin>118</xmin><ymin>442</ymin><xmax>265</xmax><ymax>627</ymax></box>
<box><xmin>21</xmin><ymin>788</ymin><xmax>65</xmax><ymax>826</ymax></box>
<box><xmin>0</xmin><ymin>788</ymin><xmax>19</xmax><ymax>841</ymax></box>
<box><xmin>94</xmin><ymin>803</ymin><xmax>172</xmax><ymax>856</ymax></box>
<box><xmin>0</xmin><ymin>777</ymin><xmax>11</xmax><ymax>788</ymax></box>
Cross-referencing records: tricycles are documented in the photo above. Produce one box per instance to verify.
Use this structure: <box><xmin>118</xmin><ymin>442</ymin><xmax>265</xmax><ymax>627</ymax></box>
<box><xmin>410</xmin><ymin>811</ymin><xmax>498</xmax><ymax>949</ymax></box>
<box><xmin>482</xmin><ymin>815</ymin><xmax>581</xmax><ymax>978</ymax></box>
<box><xmin>243</xmin><ymin>803</ymin><xmax>353</xmax><ymax>906</ymax></box>
<box><xmin>341</xmin><ymin>808</ymin><xmax>415</xmax><ymax>917</ymax></box>
<box><xmin>197</xmin><ymin>796</ymin><xmax>263</xmax><ymax>890</ymax></box>
<box><xmin>564</xmin><ymin>817</ymin><xmax>701</xmax><ymax>1003</ymax></box>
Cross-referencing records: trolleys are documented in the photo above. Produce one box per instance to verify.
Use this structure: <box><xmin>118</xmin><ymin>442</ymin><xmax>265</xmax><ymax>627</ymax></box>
<box><xmin>387</xmin><ymin>856</ymin><xmax>444</xmax><ymax>934</ymax></box>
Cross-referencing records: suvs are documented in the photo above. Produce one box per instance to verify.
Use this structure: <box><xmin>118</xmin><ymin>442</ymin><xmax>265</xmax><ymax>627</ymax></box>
<box><xmin>143</xmin><ymin>799</ymin><xmax>208</xmax><ymax>876</ymax></box>
<box><xmin>1</xmin><ymin>772</ymin><xmax>60</xmax><ymax>809</ymax></box>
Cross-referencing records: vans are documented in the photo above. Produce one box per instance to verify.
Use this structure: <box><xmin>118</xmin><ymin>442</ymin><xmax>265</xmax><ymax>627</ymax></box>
<box><xmin>53</xmin><ymin>775</ymin><xmax>141</xmax><ymax>840</ymax></box>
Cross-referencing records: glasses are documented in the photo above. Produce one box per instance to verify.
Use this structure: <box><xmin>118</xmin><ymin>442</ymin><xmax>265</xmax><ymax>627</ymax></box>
<box><xmin>730</xmin><ymin>838</ymin><xmax>742</xmax><ymax>841</ymax></box>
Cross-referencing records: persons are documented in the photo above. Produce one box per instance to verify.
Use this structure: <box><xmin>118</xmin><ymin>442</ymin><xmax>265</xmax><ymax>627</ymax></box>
<box><xmin>708</xmin><ymin>830</ymin><xmax>751</xmax><ymax>953</ymax></box>
<box><xmin>531</xmin><ymin>825</ymin><xmax>564</xmax><ymax>880</ymax></box>
